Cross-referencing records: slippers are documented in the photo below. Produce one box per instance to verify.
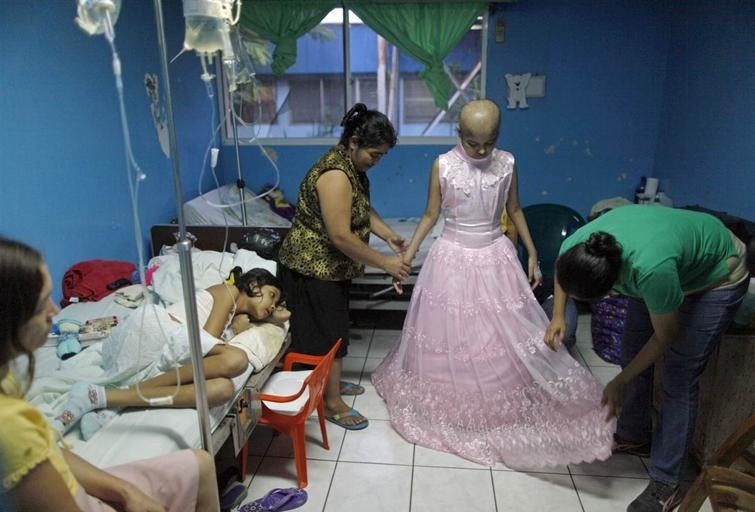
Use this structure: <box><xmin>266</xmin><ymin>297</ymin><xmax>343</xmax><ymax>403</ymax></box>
<box><xmin>339</xmin><ymin>381</ymin><xmax>364</xmax><ymax>396</ymax></box>
<box><xmin>220</xmin><ymin>468</ymin><xmax>248</xmax><ymax>509</ymax></box>
<box><xmin>325</xmin><ymin>408</ymin><xmax>368</xmax><ymax>429</ymax></box>
<box><xmin>240</xmin><ymin>488</ymin><xmax>307</xmax><ymax>512</ymax></box>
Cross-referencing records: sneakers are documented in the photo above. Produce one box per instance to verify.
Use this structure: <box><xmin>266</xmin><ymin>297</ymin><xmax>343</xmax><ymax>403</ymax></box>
<box><xmin>611</xmin><ymin>434</ymin><xmax>651</xmax><ymax>457</ymax></box>
<box><xmin>627</xmin><ymin>481</ymin><xmax>685</xmax><ymax>511</ymax></box>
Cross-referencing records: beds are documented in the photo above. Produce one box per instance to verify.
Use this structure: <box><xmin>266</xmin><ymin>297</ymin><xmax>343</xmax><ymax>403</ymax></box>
<box><xmin>176</xmin><ymin>184</ymin><xmax>438</xmax><ymax>329</ymax></box>
<box><xmin>8</xmin><ymin>226</ymin><xmax>294</xmax><ymax>469</ymax></box>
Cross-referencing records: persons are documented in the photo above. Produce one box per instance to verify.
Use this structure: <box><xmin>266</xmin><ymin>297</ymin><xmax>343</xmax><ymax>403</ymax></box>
<box><xmin>276</xmin><ymin>102</ymin><xmax>419</xmax><ymax>426</ymax></box>
<box><xmin>544</xmin><ymin>203</ymin><xmax>752</xmax><ymax>512</ymax></box>
<box><xmin>50</xmin><ymin>268</ymin><xmax>292</xmax><ymax>442</ymax></box>
<box><xmin>391</xmin><ymin>98</ymin><xmax>543</xmax><ymax>460</ymax></box>
<box><xmin>1</xmin><ymin>238</ymin><xmax>172</xmax><ymax>511</ymax></box>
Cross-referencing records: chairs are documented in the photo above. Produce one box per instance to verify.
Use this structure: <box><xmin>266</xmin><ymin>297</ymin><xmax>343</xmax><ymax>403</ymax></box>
<box><xmin>519</xmin><ymin>202</ymin><xmax>585</xmax><ymax>309</ymax></box>
<box><xmin>237</xmin><ymin>338</ymin><xmax>344</xmax><ymax>488</ymax></box>
